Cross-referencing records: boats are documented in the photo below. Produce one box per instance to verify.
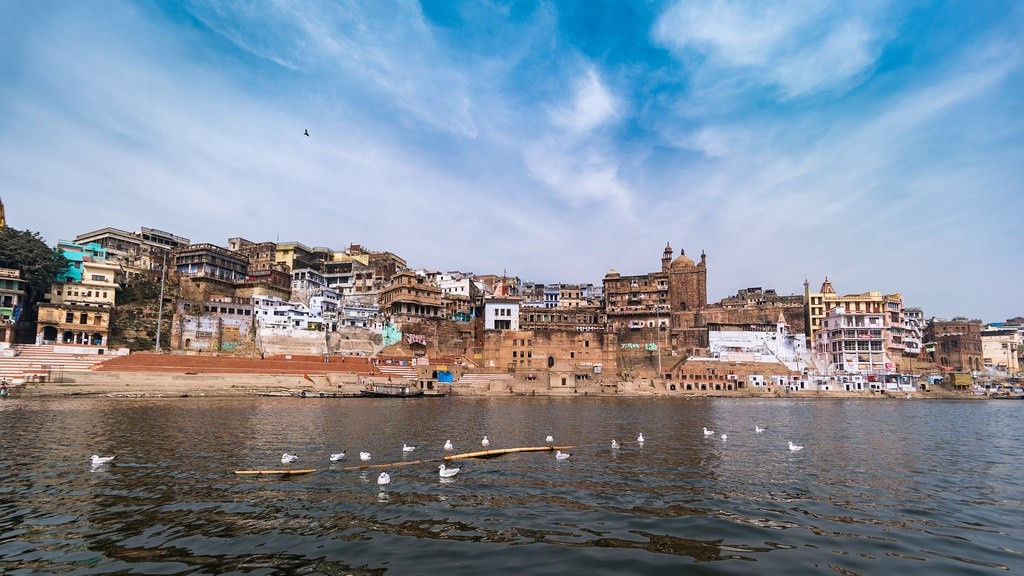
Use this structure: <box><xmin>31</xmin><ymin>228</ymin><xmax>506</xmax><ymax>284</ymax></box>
<box><xmin>990</xmin><ymin>391</ymin><xmax>1024</xmax><ymax>399</ymax></box>
<box><xmin>359</xmin><ymin>376</ymin><xmax>424</xmax><ymax>398</ymax></box>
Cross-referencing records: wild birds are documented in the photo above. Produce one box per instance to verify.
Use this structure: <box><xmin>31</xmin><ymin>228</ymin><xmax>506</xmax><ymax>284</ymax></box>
<box><xmin>402</xmin><ymin>444</ymin><xmax>418</xmax><ymax>451</ymax></box>
<box><xmin>360</xmin><ymin>451</ymin><xmax>372</xmax><ymax>461</ymax></box>
<box><xmin>555</xmin><ymin>451</ymin><xmax>573</xmax><ymax>460</ymax></box>
<box><xmin>89</xmin><ymin>454</ymin><xmax>120</xmax><ymax>463</ymax></box>
<box><xmin>303</xmin><ymin>128</ymin><xmax>309</xmax><ymax>137</ymax></box>
<box><xmin>611</xmin><ymin>439</ymin><xmax>622</xmax><ymax>448</ymax></box>
<box><xmin>787</xmin><ymin>441</ymin><xmax>804</xmax><ymax>450</ymax></box>
<box><xmin>377</xmin><ymin>472</ymin><xmax>390</xmax><ymax>484</ymax></box>
<box><xmin>281</xmin><ymin>453</ymin><xmax>300</xmax><ymax>472</ymax></box>
<box><xmin>702</xmin><ymin>427</ymin><xmax>714</xmax><ymax>434</ymax></box>
<box><xmin>754</xmin><ymin>425</ymin><xmax>771</xmax><ymax>432</ymax></box>
<box><xmin>637</xmin><ymin>432</ymin><xmax>644</xmax><ymax>441</ymax></box>
<box><xmin>546</xmin><ymin>435</ymin><xmax>554</xmax><ymax>442</ymax></box>
<box><xmin>720</xmin><ymin>433</ymin><xmax>727</xmax><ymax>438</ymax></box>
<box><xmin>481</xmin><ymin>436</ymin><xmax>490</xmax><ymax>447</ymax></box>
<box><xmin>330</xmin><ymin>449</ymin><xmax>349</xmax><ymax>460</ymax></box>
<box><xmin>444</xmin><ymin>440</ymin><xmax>453</xmax><ymax>451</ymax></box>
<box><xmin>438</xmin><ymin>463</ymin><xmax>464</xmax><ymax>478</ymax></box>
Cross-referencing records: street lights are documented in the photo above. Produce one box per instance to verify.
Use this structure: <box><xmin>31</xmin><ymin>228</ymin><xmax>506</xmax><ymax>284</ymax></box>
<box><xmin>156</xmin><ymin>248</ymin><xmax>171</xmax><ymax>351</ymax></box>
